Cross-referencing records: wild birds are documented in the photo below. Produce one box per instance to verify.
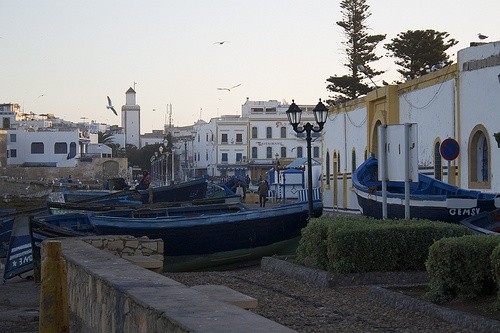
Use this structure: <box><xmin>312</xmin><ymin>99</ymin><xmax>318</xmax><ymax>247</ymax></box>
<box><xmin>213</xmin><ymin>41</ymin><xmax>234</xmax><ymax>45</ymax></box>
<box><xmin>104</xmin><ymin>96</ymin><xmax>118</xmax><ymax>116</ymax></box>
<box><xmin>217</xmin><ymin>84</ymin><xmax>242</xmax><ymax>91</ymax></box>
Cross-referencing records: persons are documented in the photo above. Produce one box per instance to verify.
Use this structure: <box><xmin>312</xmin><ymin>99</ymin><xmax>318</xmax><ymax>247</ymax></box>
<box><xmin>223</xmin><ymin>174</ymin><xmax>269</xmax><ymax>208</ymax></box>
<box><xmin>67</xmin><ymin>174</ymin><xmax>72</xmax><ymax>183</ymax></box>
<box><xmin>59</xmin><ymin>176</ymin><xmax>64</xmax><ymax>183</ymax></box>
<box><xmin>139</xmin><ymin>171</ymin><xmax>150</xmax><ymax>190</ymax></box>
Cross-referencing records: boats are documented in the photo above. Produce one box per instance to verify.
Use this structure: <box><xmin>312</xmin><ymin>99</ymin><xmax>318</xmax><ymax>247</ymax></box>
<box><xmin>0</xmin><ymin>176</ymin><xmax>324</xmax><ymax>280</ymax></box>
<box><xmin>351</xmin><ymin>155</ymin><xmax>500</xmax><ymax>222</ymax></box>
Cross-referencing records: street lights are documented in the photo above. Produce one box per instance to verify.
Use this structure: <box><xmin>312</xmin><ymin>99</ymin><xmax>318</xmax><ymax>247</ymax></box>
<box><xmin>284</xmin><ymin>97</ymin><xmax>328</xmax><ymax>219</ymax></box>
